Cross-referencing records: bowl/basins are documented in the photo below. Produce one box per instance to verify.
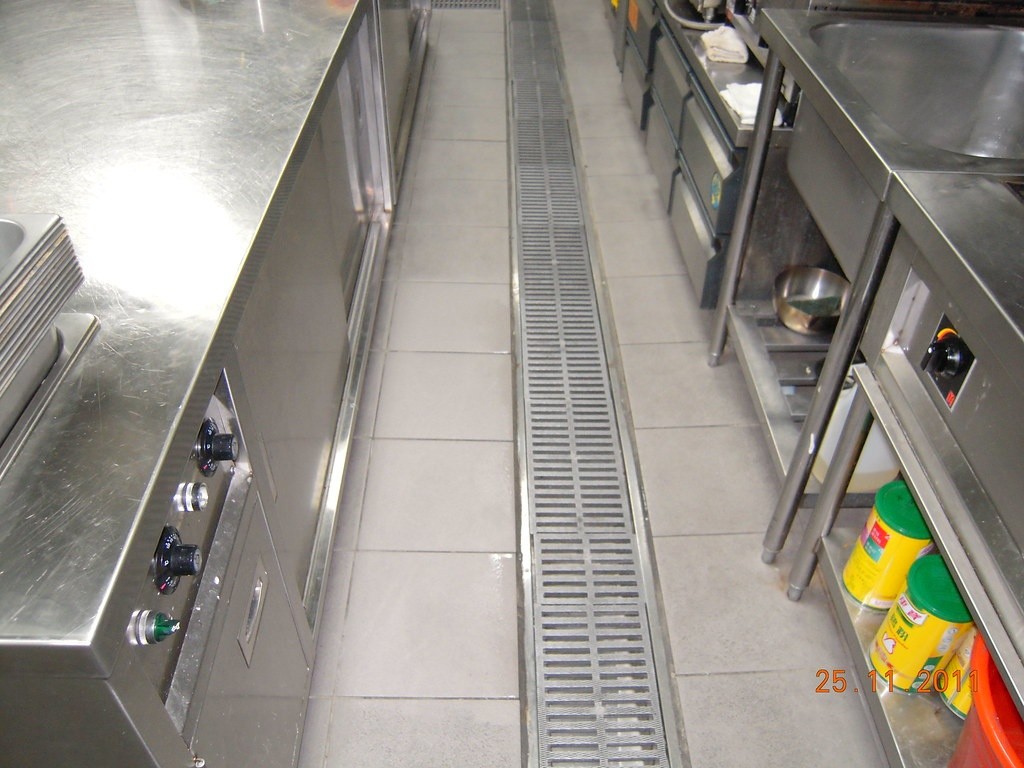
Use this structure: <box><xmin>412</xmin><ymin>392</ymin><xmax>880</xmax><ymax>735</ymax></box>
<box><xmin>773</xmin><ymin>267</ymin><xmax>852</xmax><ymax>336</ymax></box>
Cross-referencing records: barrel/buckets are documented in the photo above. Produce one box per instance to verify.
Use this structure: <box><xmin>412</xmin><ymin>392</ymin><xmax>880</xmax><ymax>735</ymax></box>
<box><xmin>947</xmin><ymin>632</ymin><xmax>1024</xmax><ymax>768</ymax></box>
